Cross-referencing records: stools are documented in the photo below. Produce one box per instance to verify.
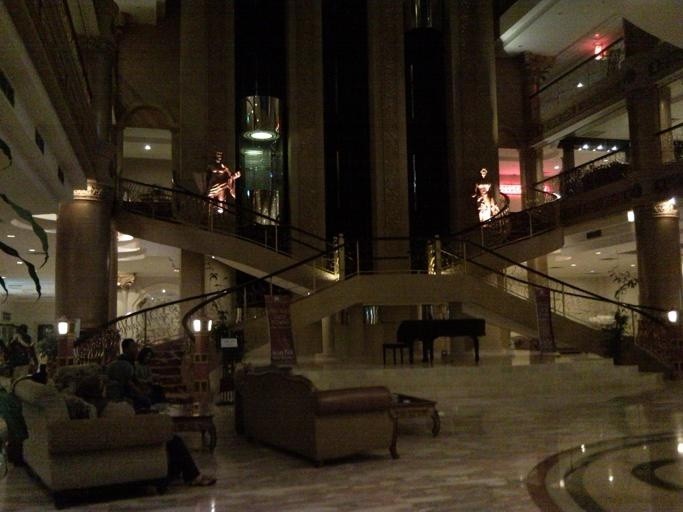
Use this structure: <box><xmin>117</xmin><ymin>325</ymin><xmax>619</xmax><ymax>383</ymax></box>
<box><xmin>382</xmin><ymin>342</ymin><xmax>401</xmax><ymax>364</ymax></box>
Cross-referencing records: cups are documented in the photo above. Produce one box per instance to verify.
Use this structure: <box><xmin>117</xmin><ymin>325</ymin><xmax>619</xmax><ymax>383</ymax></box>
<box><xmin>193</xmin><ymin>400</ymin><xmax>200</xmax><ymax>416</ymax></box>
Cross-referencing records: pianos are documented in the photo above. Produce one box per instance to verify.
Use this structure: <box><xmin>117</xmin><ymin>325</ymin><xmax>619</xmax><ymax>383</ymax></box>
<box><xmin>396</xmin><ymin>318</ymin><xmax>485</xmax><ymax>365</ymax></box>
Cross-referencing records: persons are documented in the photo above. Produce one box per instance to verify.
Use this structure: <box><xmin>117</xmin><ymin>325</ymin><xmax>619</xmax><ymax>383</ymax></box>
<box><xmin>205</xmin><ymin>151</ymin><xmax>242</xmax><ymax>215</ymax></box>
<box><xmin>0</xmin><ymin>324</ymin><xmax>216</xmax><ymax>488</ymax></box>
<box><xmin>472</xmin><ymin>168</ymin><xmax>495</xmax><ymax>228</ymax></box>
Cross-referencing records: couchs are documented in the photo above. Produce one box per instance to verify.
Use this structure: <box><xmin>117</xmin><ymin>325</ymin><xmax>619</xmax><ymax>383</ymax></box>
<box><xmin>0</xmin><ymin>376</ymin><xmax>28</xmax><ymax>463</ymax></box>
<box><xmin>56</xmin><ymin>362</ymin><xmax>102</xmax><ymax>381</ymax></box>
<box><xmin>13</xmin><ymin>379</ymin><xmax>173</xmax><ymax>508</ymax></box>
<box><xmin>235</xmin><ymin>371</ymin><xmax>399</xmax><ymax>466</ymax></box>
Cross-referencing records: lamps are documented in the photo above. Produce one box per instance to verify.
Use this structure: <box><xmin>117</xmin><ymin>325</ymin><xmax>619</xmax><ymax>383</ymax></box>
<box><xmin>53</xmin><ymin>314</ymin><xmax>77</xmax><ymax>339</ymax></box>
<box><xmin>243</xmin><ymin>94</ymin><xmax>280</xmax><ymax>143</ymax></box>
<box><xmin>592</xmin><ymin>33</ymin><xmax>605</xmax><ymax>63</ymax></box>
<box><xmin>191</xmin><ymin>306</ymin><xmax>213</xmax><ymax>337</ymax></box>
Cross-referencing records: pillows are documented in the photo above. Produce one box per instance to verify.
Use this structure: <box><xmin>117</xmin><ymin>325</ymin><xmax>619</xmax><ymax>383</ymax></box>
<box><xmin>64</xmin><ymin>394</ymin><xmax>97</xmax><ymax>418</ymax></box>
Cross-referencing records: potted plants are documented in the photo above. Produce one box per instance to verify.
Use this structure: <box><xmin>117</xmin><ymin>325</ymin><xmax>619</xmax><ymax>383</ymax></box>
<box><xmin>601</xmin><ymin>265</ymin><xmax>638</xmax><ymax>342</ymax></box>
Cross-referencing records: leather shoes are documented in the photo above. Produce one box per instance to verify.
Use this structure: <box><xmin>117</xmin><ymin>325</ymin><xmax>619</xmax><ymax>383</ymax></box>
<box><xmin>186</xmin><ymin>476</ymin><xmax>217</xmax><ymax>487</ymax></box>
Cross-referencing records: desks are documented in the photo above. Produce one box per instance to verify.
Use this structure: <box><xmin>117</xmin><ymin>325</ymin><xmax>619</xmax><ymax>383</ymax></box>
<box><xmin>158</xmin><ymin>403</ymin><xmax>218</xmax><ymax>454</ymax></box>
<box><xmin>393</xmin><ymin>391</ymin><xmax>440</xmax><ymax>438</ymax></box>
